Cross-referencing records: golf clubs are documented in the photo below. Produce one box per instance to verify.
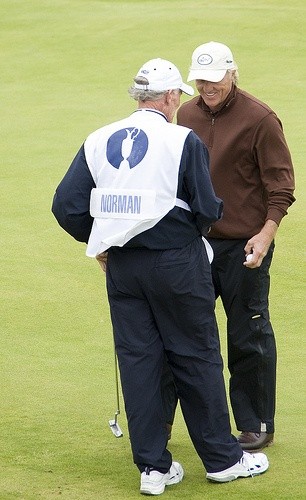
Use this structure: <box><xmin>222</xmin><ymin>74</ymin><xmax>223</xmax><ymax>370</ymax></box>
<box><xmin>108</xmin><ymin>348</ymin><xmax>124</xmax><ymax>439</ymax></box>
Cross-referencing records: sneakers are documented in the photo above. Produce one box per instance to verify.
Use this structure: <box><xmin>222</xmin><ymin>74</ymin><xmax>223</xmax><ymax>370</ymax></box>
<box><xmin>205</xmin><ymin>450</ymin><xmax>269</xmax><ymax>482</ymax></box>
<box><xmin>139</xmin><ymin>462</ymin><xmax>184</xmax><ymax>494</ymax></box>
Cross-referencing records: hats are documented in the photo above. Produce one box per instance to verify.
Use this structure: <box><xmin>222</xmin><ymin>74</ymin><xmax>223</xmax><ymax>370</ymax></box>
<box><xmin>187</xmin><ymin>41</ymin><xmax>235</xmax><ymax>82</ymax></box>
<box><xmin>134</xmin><ymin>57</ymin><xmax>194</xmax><ymax>96</ymax></box>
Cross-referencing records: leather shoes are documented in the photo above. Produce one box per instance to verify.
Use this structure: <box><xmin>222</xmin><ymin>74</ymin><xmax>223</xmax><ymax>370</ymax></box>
<box><xmin>167</xmin><ymin>424</ymin><xmax>172</xmax><ymax>440</ymax></box>
<box><xmin>237</xmin><ymin>431</ymin><xmax>274</xmax><ymax>452</ymax></box>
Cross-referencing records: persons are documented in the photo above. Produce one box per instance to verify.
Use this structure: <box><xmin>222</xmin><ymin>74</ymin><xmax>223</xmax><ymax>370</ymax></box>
<box><xmin>177</xmin><ymin>41</ymin><xmax>296</xmax><ymax>453</ymax></box>
<box><xmin>51</xmin><ymin>58</ymin><xmax>270</xmax><ymax>495</ymax></box>
